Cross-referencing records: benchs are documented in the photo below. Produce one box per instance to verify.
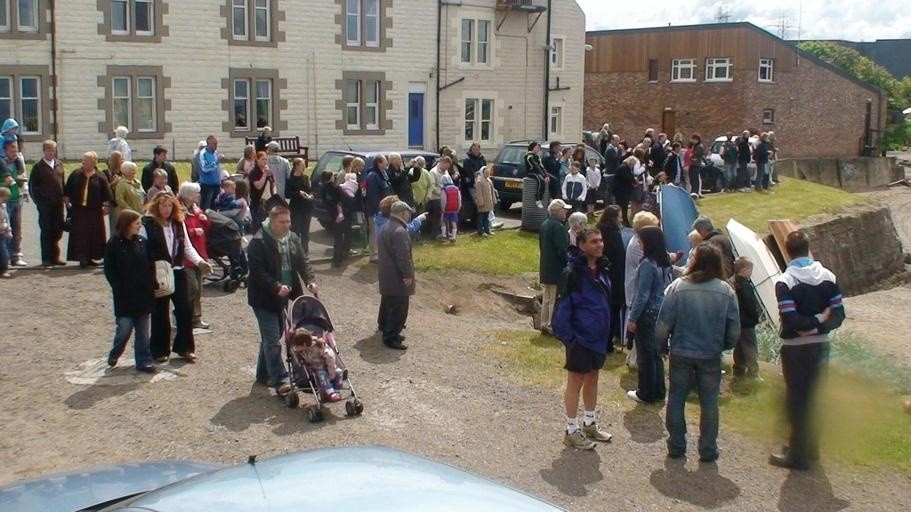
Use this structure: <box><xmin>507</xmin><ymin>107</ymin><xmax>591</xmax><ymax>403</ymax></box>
<box><xmin>244</xmin><ymin>135</ymin><xmax>310</xmax><ymax>169</ymax></box>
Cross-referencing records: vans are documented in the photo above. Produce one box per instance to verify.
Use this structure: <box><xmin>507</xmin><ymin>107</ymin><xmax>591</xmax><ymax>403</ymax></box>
<box><xmin>0</xmin><ymin>449</ymin><xmax>569</xmax><ymax>512</ymax></box>
<box><xmin>307</xmin><ymin>149</ymin><xmax>477</xmax><ymax>239</ymax></box>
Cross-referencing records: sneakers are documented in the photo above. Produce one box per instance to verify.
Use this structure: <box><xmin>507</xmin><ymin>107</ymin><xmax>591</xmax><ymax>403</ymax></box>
<box><xmin>155</xmin><ymin>354</ymin><xmax>169</xmax><ymax>362</ymax></box>
<box><xmin>184</xmin><ymin>351</ymin><xmax>197</xmax><ymax>359</ymax></box>
<box><xmin>277</xmin><ymin>383</ymin><xmax>292</xmax><ymax>395</ymax></box>
<box><xmin>324</xmin><ymin>392</ymin><xmax>341</xmax><ymax>402</ymax></box>
<box><xmin>564</xmin><ymin>428</ymin><xmax>597</xmax><ymax>450</ymax></box>
<box><xmin>346</xmin><ymin>249</ymin><xmax>357</xmax><ymax>254</ymax></box>
<box><xmin>108</xmin><ymin>351</ymin><xmax>117</xmax><ymax>366</ymax></box>
<box><xmin>627</xmin><ymin>390</ymin><xmax>644</xmax><ymax>402</ymax></box>
<box><xmin>582</xmin><ymin>421</ymin><xmax>612</xmax><ymax>441</ymax></box>
<box><xmin>193</xmin><ymin>321</ymin><xmax>210</xmax><ymax>329</ymax></box>
<box><xmin>138</xmin><ymin>363</ymin><xmax>155</xmax><ymax>373</ymax></box>
<box><xmin>335</xmin><ymin>215</ymin><xmax>344</xmax><ymax>223</ymax></box>
<box><xmin>11</xmin><ymin>259</ymin><xmax>27</xmax><ymax>266</ymax></box>
<box><xmin>386</xmin><ymin>334</ymin><xmax>408</xmax><ymax>349</ymax></box>
<box><xmin>769</xmin><ymin>452</ymin><xmax>791</xmax><ymax>467</ymax></box>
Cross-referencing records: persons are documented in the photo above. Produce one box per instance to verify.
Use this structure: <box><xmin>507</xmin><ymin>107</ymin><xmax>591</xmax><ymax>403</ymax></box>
<box><xmin>540</xmin><ymin>199</ymin><xmax>846</xmax><ymax>470</ymax></box>
<box><xmin>0</xmin><ymin>118</ymin><xmax>499</xmax><ymax>292</ymax></box>
<box><xmin>102</xmin><ymin>179</ymin><xmax>212</xmax><ymax>376</ymax></box>
<box><xmin>292</xmin><ymin>328</ymin><xmax>341</xmax><ymax>400</ymax></box>
<box><xmin>245</xmin><ymin>207</ymin><xmax>319</xmax><ymax>393</ymax></box>
<box><xmin>374</xmin><ymin>194</ymin><xmax>429</xmax><ymax>350</ymax></box>
<box><xmin>523</xmin><ymin>123</ymin><xmax>782</xmax><ymax>216</ymax></box>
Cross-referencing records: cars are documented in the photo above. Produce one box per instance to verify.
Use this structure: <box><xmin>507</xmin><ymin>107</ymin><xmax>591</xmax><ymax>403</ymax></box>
<box><xmin>489</xmin><ymin>139</ymin><xmax>606</xmax><ymax>212</ymax></box>
<box><xmin>665</xmin><ymin>145</ymin><xmax>727</xmax><ymax>194</ymax></box>
<box><xmin>704</xmin><ymin>134</ymin><xmax>769</xmax><ymax>183</ymax></box>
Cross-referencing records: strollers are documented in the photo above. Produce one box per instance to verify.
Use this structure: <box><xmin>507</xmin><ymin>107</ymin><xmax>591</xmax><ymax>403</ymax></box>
<box><xmin>201</xmin><ymin>208</ymin><xmax>250</xmax><ymax>293</ymax></box>
<box><xmin>276</xmin><ymin>283</ymin><xmax>364</xmax><ymax>424</ymax></box>
<box><xmin>220</xmin><ymin>173</ymin><xmax>259</xmax><ymax>233</ymax></box>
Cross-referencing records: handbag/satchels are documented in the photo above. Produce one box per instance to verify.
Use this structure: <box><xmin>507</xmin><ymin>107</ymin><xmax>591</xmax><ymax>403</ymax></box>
<box><xmin>153</xmin><ymin>260</ymin><xmax>175</xmax><ymax>299</ymax></box>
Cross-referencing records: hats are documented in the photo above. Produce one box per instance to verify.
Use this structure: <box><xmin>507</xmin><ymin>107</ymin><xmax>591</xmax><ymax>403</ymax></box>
<box><xmin>391</xmin><ymin>201</ymin><xmax>413</xmax><ymax>214</ymax></box>
<box><xmin>265</xmin><ymin>141</ymin><xmax>280</xmax><ymax>151</ymax></box>
<box><xmin>547</xmin><ymin>199</ymin><xmax>573</xmax><ymax>210</ymax></box>
